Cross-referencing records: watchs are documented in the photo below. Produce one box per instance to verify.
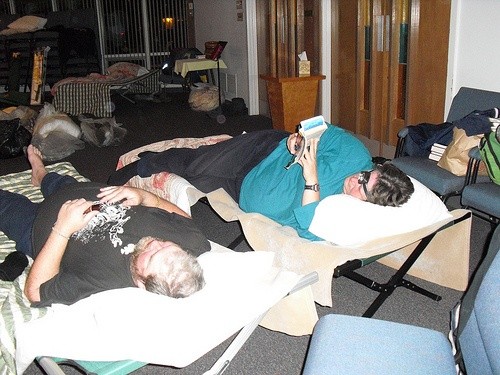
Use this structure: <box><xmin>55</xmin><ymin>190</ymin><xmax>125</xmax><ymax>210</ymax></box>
<box><xmin>304</xmin><ymin>184</ymin><xmax>320</xmax><ymax>192</ymax></box>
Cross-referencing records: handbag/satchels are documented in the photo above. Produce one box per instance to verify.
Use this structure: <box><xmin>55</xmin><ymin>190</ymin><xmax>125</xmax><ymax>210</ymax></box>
<box><xmin>188</xmin><ymin>83</ymin><xmax>225</xmax><ymax>112</ymax></box>
<box><xmin>438</xmin><ymin>126</ymin><xmax>488</xmax><ymax>178</ymax></box>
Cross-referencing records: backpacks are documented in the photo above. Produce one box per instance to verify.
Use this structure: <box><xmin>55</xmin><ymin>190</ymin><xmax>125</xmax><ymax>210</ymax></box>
<box><xmin>478</xmin><ymin>132</ymin><xmax>500</xmax><ymax>186</ymax></box>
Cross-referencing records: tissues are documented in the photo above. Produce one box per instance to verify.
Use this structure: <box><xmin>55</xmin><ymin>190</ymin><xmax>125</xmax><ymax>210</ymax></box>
<box><xmin>297</xmin><ymin>51</ymin><xmax>310</xmax><ymax>77</ymax></box>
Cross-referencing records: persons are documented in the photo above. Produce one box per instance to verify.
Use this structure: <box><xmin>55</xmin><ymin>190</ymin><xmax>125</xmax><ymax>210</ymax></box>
<box><xmin>108</xmin><ymin>119</ymin><xmax>415</xmax><ymax>241</ymax></box>
<box><xmin>0</xmin><ymin>145</ymin><xmax>211</xmax><ymax>308</ymax></box>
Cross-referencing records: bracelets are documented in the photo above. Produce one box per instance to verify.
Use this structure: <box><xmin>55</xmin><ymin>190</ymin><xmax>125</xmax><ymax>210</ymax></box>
<box><xmin>155</xmin><ymin>195</ymin><xmax>161</xmax><ymax>208</ymax></box>
<box><xmin>52</xmin><ymin>227</ymin><xmax>71</xmax><ymax>241</ymax></box>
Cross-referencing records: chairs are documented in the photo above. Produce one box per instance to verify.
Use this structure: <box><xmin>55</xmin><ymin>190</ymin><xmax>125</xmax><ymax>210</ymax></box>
<box><xmin>458</xmin><ymin>123</ymin><xmax>500</xmax><ymax>227</ymax></box>
<box><xmin>391</xmin><ymin>87</ymin><xmax>500</xmax><ymax>203</ymax></box>
<box><xmin>301</xmin><ymin>224</ymin><xmax>500</xmax><ymax>375</ymax></box>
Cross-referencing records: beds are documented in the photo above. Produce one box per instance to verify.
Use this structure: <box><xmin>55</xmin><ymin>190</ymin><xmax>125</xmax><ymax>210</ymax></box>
<box><xmin>0</xmin><ymin>134</ymin><xmax>473</xmax><ymax>375</ymax></box>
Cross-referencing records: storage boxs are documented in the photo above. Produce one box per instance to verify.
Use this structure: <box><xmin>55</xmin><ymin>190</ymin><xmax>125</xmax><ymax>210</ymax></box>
<box><xmin>28</xmin><ymin>47</ymin><xmax>51</xmax><ymax>107</ymax></box>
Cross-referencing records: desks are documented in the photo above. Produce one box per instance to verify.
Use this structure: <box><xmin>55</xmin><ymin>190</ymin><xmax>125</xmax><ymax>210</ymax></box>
<box><xmin>174</xmin><ymin>55</ymin><xmax>228</xmax><ymax>78</ymax></box>
<box><xmin>260</xmin><ymin>70</ymin><xmax>326</xmax><ymax>132</ymax></box>
<box><xmin>55</xmin><ymin>68</ymin><xmax>161</xmax><ymax>116</ymax></box>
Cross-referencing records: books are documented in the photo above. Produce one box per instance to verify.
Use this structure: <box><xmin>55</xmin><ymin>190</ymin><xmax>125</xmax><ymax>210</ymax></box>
<box><xmin>428</xmin><ymin>143</ymin><xmax>448</xmax><ymax>161</ymax></box>
<box><xmin>286</xmin><ymin>115</ymin><xmax>328</xmax><ymax>170</ymax></box>
